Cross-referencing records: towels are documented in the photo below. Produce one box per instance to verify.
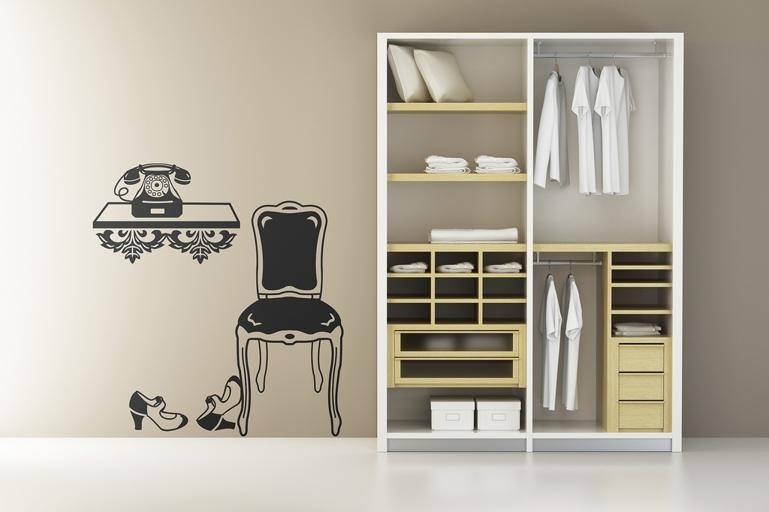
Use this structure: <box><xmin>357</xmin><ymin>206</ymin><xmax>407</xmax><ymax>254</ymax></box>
<box><xmin>614</xmin><ymin>323</ymin><xmax>664</xmax><ymax>338</ymax></box>
<box><xmin>388</xmin><ymin>227</ymin><xmax>525</xmax><ymax>275</ymax></box>
<box><xmin>422</xmin><ymin>153</ymin><xmax>521</xmax><ymax>175</ymax></box>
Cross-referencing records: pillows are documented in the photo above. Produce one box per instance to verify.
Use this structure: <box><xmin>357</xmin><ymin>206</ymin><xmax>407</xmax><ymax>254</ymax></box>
<box><xmin>386</xmin><ymin>41</ymin><xmax>472</xmax><ymax>103</ymax></box>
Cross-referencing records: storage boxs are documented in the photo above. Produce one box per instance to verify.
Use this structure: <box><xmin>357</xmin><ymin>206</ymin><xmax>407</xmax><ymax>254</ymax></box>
<box><xmin>430</xmin><ymin>397</ymin><xmax>522</xmax><ymax>432</ymax></box>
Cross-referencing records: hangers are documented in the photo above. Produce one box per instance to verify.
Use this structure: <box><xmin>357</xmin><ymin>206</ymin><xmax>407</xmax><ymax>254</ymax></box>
<box><xmin>547</xmin><ymin>260</ymin><xmax>576</xmax><ymax>282</ymax></box>
<box><xmin>548</xmin><ymin>49</ymin><xmax>624</xmax><ymax>82</ymax></box>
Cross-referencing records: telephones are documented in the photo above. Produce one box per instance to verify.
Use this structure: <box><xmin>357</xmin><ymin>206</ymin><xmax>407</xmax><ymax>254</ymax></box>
<box><xmin>113</xmin><ymin>163</ymin><xmax>191</xmax><ymax>217</ymax></box>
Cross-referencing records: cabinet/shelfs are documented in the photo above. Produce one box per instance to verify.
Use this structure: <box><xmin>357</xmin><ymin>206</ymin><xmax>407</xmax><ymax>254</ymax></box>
<box><xmin>376</xmin><ymin>32</ymin><xmax>685</xmax><ymax>460</ymax></box>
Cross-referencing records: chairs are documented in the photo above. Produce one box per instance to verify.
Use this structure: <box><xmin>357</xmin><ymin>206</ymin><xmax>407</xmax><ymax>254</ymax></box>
<box><xmin>233</xmin><ymin>199</ymin><xmax>345</xmax><ymax>439</ymax></box>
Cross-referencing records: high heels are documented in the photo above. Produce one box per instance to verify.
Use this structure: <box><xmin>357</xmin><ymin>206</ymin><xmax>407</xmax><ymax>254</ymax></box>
<box><xmin>128</xmin><ymin>390</ymin><xmax>189</xmax><ymax>432</ymax></box>
<box><xmin>196</xmin><ymin>375</ymin><xmax>243</xmax><ymax>433</ymax></box>
